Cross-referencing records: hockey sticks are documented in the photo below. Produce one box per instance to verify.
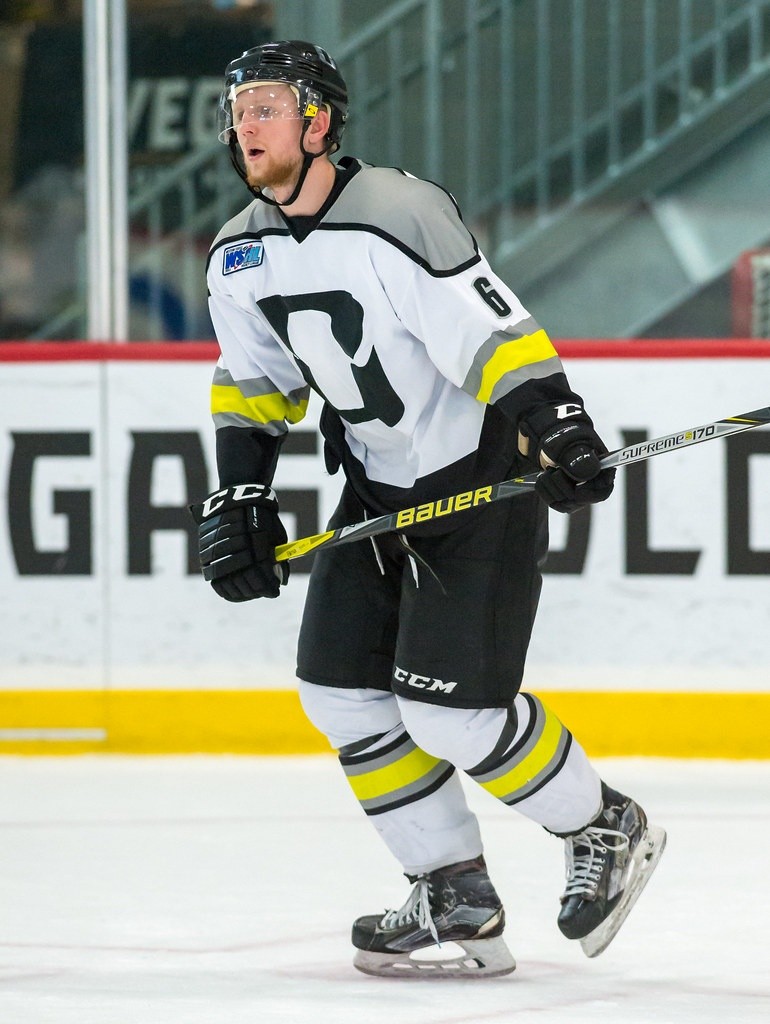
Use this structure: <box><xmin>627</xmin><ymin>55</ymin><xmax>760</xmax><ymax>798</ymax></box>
<box><xmin>272</xmin><ymin>408</ymin><xmax>770</xmax><ymax>563</ymax></box>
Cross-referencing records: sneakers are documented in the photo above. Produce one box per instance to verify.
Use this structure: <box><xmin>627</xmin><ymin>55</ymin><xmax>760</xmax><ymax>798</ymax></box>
<box><xmin>543</xmin><ymin>779</ymin><xmax>667</xmax><ymax>958</ymax></box>
<box><xmin>352</xmin><ymin>853</ymin><xmax>516</xmax><ymax>980</ymax></box>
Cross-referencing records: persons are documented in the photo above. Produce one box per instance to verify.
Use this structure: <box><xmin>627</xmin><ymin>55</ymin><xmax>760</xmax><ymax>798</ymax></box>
<box><xmin>190</xmin><ymin>41</ymin><xmax>667</xmax><ymax>977</ymax></box>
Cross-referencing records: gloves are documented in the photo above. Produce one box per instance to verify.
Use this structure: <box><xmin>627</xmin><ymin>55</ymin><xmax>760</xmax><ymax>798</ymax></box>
<box><xmin>521</xmin><ymin>403</ymin><xmax>616</xmax><ymax>512</ymax></box>
<box><xmin>189</xmin><ymin>484</ymin><xmax>290</xmax><ymax>603</ymax></box>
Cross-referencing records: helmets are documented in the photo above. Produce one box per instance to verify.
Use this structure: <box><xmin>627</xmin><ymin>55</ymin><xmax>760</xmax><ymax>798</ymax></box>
<box><xmin>225</xmin><ymin>40</ymin><xmax>351</xmax><ymax>143</ymax></box>
